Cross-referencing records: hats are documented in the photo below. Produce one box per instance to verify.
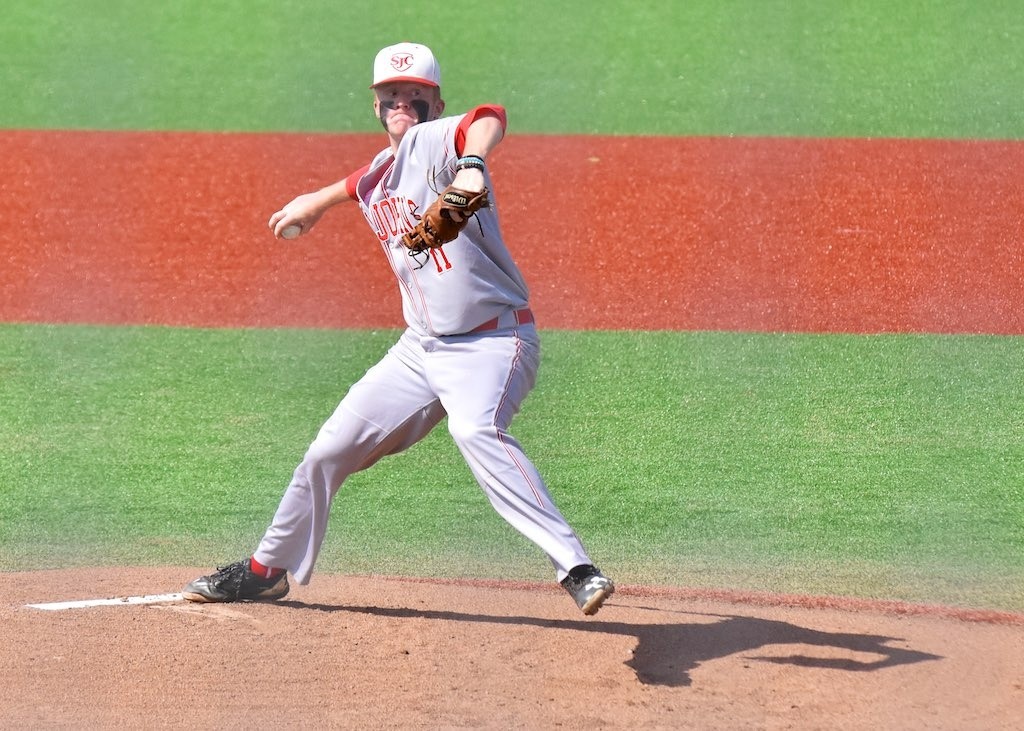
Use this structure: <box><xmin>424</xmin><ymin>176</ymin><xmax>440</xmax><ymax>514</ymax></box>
<box><xmin>368</xmin><ymin>42</ymin><xmax>440</xmax><ymax>90</ymax></box>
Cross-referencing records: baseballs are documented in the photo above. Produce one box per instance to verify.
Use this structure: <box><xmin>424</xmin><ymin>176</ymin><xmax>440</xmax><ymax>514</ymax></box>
<box><xmin>281</xmin><ymin>223</ymin><xmax>302</xmax><ymax>239</ymax></box>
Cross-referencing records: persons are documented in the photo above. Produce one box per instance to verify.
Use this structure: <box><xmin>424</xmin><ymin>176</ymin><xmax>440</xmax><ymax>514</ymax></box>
<box><xmin>179</xmin><ymin>42</ymin><xmax>615</xmax><ymax>616</ymax></box>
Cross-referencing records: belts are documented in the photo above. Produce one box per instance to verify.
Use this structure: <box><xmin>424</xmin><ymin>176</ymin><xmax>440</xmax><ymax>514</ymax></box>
<box><xmin>471</xmin><ymin>309</ymin><xmax>534</xmax><ymax>332</ymax></box>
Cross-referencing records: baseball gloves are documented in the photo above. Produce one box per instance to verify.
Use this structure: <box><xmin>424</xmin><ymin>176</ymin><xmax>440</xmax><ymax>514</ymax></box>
<box><xmin>400</xmin><ymin>186</ymin><xmax>496</xmax><ymax>271</ymax></box>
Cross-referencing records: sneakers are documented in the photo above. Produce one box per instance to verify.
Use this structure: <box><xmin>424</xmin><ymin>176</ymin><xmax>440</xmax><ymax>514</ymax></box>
<box><xmin>181</xmin><ymin>558</ymin><xmax>290</xmax><ymax>603</ymax></box>
<box><xmin>560</xmin><ymin>563</ymin><xmax>615</xmax><ymax>616</ymax></box>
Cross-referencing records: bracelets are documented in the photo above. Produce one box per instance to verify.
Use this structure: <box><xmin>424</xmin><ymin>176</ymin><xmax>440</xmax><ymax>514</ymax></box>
<box><xmin>454</xmin><ymin>155</ymin><xmax>485</xmax><ymax>173</ymax></box>
